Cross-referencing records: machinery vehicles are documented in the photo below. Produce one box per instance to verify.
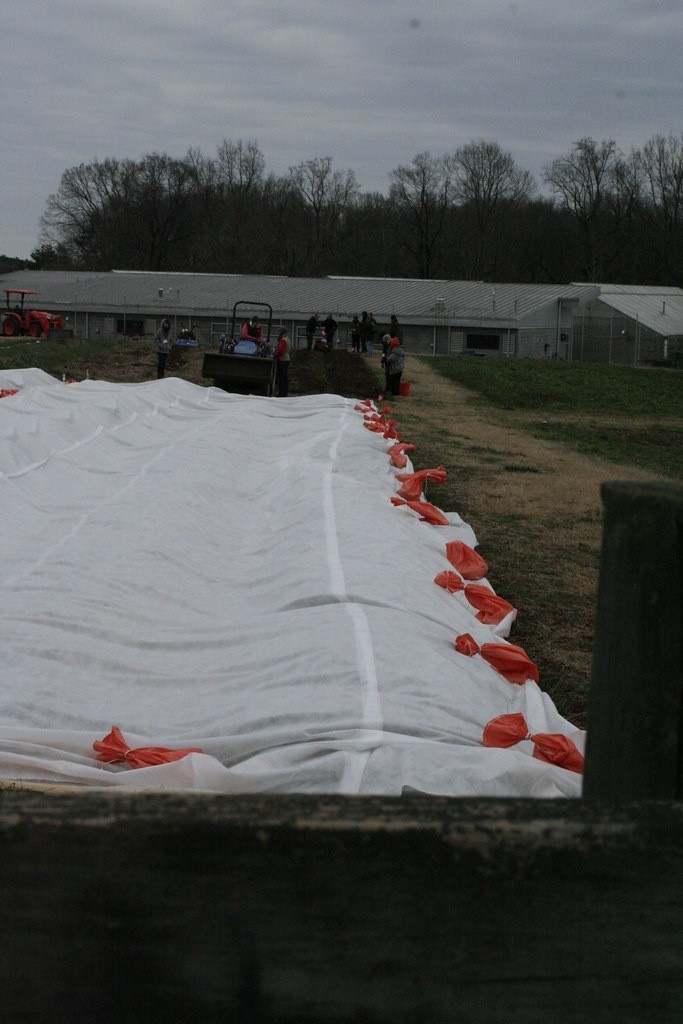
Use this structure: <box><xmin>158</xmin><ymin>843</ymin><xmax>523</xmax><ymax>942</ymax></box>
<box><xmin>201</xmin><ymin>300</ymin><xmax>278</xmax><ymax>397</ymax></box>
<box><xmin>3</xmin><ymin>289</ymin><xmax>73</xmax><ymax>340</ymax></box>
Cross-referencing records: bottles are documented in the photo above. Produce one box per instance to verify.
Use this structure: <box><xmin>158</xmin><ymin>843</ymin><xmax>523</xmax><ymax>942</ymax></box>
<box><xmin>62</xmin><ymin>365</ymin><xmax>68</xmax><ymax>382</ymax></box>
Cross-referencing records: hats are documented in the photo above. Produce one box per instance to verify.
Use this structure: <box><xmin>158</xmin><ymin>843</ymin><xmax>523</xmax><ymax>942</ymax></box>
<box><xmin>390</xmin><ymin>336</ymin><xmax>400</xmax><ymax>345</ymax></box>
<box><xmin>383</xmin><ymin>334</ymin><xmax>391</xmax><ymax>342</ymax></box>
<box><xmin>279</xmin><ymin>326</ymin><xmax>289</xmax><ymax>334</ymax></box>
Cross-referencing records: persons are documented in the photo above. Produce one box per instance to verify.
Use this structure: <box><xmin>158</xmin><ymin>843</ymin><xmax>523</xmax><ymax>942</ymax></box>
<box><xmin>14</xmin><ymin>305</ymin><xmax>26</xmax><ymax>321</ymax></box>
<box><xmin>272</xmin><ymin>327</ymin><xmax>291</xmax><ymax>397</ymax></box>
<box><xmin>242</xmin><ymin>316</ymin><xmax>264</xmax><ymax>352</ymax></box>
<box><xmin>153</xmin><ymin>319</ymin><xmax>173</xmax><ymax>379</ymax></box>
<box><xmin>352</xmin><ymin>311</ymin><xmax>378</xmax><ymax>357</ymax></box>
<box><xmin>389</xmin><ymin>314</ymin><xmax>404</xmax><ymax>346</ymax></box>
<box><xmin>306</xmin><ymin>314</ymin><xmax>320</xmax><ymax>349</ymax></box>
<box><xmin>381</xmin><ymin>335</ymin><xmax>405</xmax><ymax>395</ymax></box>
<box><xmin>323</xmin><ymin>313</ymin><xmax>338</xmax><ymax>348</ymax></box>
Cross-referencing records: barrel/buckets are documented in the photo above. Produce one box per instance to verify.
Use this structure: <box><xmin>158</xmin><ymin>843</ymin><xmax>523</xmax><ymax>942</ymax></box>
<box><xmin>400</xmin><ymin>381</ymin><xmax>410</xmax><ymax>397</ymax></box>
